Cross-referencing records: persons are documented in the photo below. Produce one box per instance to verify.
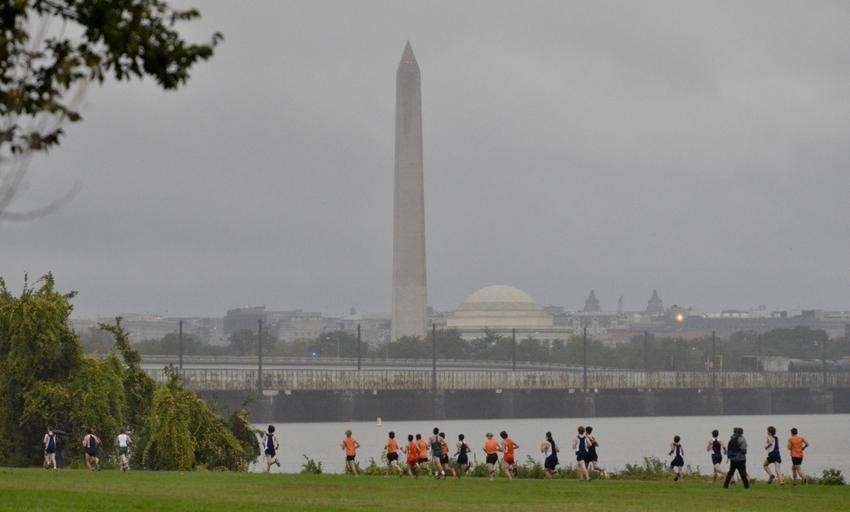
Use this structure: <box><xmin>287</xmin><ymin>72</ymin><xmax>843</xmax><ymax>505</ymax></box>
<box><xmin>572</xmin><ymin>426</ymin><xmax>607</xmax><ymax>481</ymax></box>
<box><xmin>707</xmin><ymin>427</ymin><xmax>750</xmax><ymax>489</ymax></box>
<box><xmin>339</xmin><ymin>430</ymin><xmax>360</xmax><ymax>478</ymax></box>
<box><xmin>261</xmin><ymin>424</ymin><xmax>280</xmax><ymax>474</ymax></box>
<box><xmin>788</xmin><ymin>428</ymin><xmax>808</xmax><ymax>485</ymax></box>
<box><xmin>669</xmin><ymin>435</ymin><xmax>685</xmax><ymax>482</ymax></box>
<box><xmin>43</xmin><ymin>426</ymin><xmax>58</xmax><ymax>470</ymax></box>
<box><xmin>762</xmin><ymin>426</ymin><xmax>785</xmax><ymax>486</ymax></box>
<box><xmin>81</xmin><ymin>428</ymin><xmax>102</xmax><ymax>471</ymax></box>
<box><xmin>485</xmin><ymin>431</ymin><xmax>519</xmax><ymax>480</ymax></box>
<box><xmin>540</xmin><ymin>432</ymin><xmax>559</xmax><ymax>481</ymax></box>
<box><xmin>113</xmin><ymin>427</ymin><xmax>133</xmax><ymax>471</ymax></box>
<box><xmin>383</xmin><ymin>427</ymin><xmax>471</xmax><ymax>482</ymax></box>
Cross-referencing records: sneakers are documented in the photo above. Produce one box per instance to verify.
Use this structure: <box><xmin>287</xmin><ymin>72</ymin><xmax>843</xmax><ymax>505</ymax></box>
<box><xmin>767</xmin><ymin>474</ymin><xmax>775</xmax><ymax>484</ymax></box>
<box><xmin>779</xmin><ymin>482</ymin><xmax>785</xmax><ymax>487</ymax></box>
<box><xmin>673</xmin><ymin>472</ymin><xmax>680</xmax><ymax>481</ymax></box>
<box><xmin>512</xmin><ymin>467</ymin><xmax>518</xmax><ymax>477</ymax></box>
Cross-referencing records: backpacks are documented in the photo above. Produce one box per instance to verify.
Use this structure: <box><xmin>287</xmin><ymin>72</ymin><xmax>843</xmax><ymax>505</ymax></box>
<box><xmin>726</xmin><ymin>440</ymin><xmax>739</xmax><ymax>458</ymax></box>
